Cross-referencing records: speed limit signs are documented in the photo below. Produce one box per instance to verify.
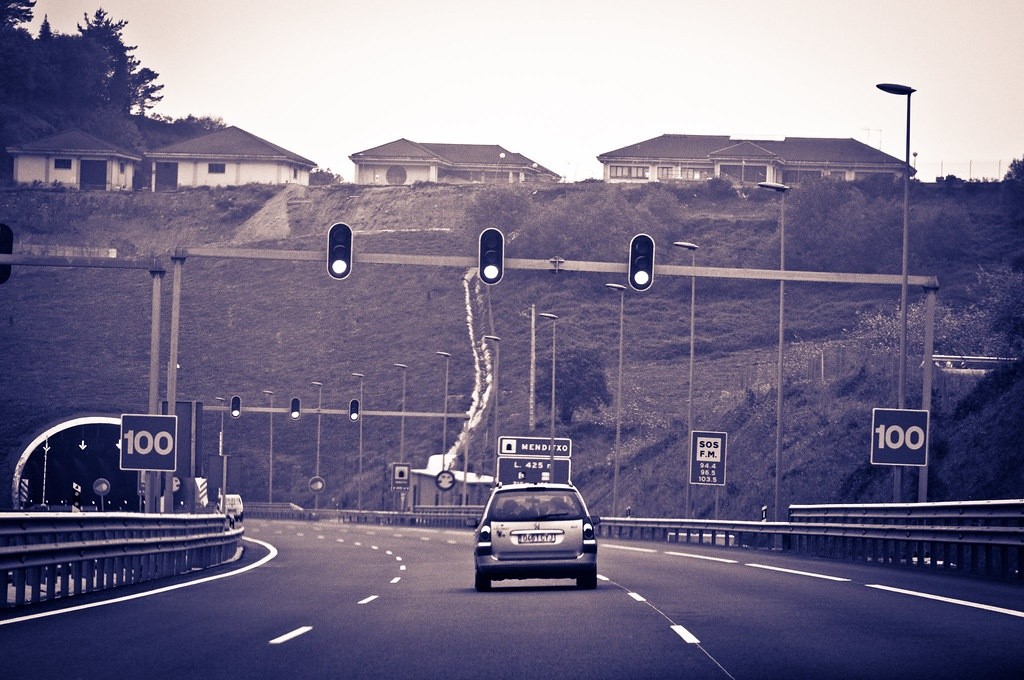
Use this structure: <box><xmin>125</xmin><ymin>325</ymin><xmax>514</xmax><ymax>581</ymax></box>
<box><xmin>119</xmin><ymin>410</ymin><xmax>178</xmax><ymax>475</ymax></box>
<box><xmin>868</xmin><ymin>405</ymin><xmax>930</xmax><ymax>471</ymax></box>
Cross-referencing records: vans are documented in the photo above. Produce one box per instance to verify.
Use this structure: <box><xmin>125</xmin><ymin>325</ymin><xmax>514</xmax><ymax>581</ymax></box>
<box><xmin>220</xmin><ymin>494</ymin><xmax>244</xmax><ymax>522</ymax></box>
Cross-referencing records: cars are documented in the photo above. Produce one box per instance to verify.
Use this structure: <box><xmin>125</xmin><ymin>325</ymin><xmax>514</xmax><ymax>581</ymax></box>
<box><xmin>473</xmin><ymin>483</ymin><xmax>602</xmax><ymax>592</ymax></box>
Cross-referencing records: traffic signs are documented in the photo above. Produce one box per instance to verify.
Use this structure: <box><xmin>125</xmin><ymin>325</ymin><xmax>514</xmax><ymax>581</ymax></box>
<box><xmin>496</xmin><ymin>457</ymin><xmax>572</xmax><ymax>485</ymax></box>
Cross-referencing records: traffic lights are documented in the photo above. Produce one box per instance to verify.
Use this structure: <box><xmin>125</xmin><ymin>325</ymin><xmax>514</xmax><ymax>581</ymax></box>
<box><xmin>322</xmin><ymin>221</ymin><xmax>355</xmax><ymax>280</ymax></box>
<box><xmin>628</xmin><ymin>231</ymin><xmax>656</xmax><ymax>292</ymax></box>
<box><xmin>476</xmin><ymin>225</ymin><xmax>506</xmax><ymax>285</ymax></box>
<box><xmin>349</xmin><ymin>397</ymin><xmax>360</xmax><ymax>423</ymax></box>
<box><xmin>229</xmin><ymin>394</ymin><xmax>242</xmax><ymax>419</ymax></box>
<box><xmin>289</xmin><ymin>397</ymin><xmax>302</xmax><ymax>422</ymax></box>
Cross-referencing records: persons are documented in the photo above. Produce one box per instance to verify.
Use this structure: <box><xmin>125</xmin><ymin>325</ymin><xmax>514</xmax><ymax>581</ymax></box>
<box><xmin>497</xmin><ymin>496</ymin><xmax>572</xmax><ymax>521</ymax></box>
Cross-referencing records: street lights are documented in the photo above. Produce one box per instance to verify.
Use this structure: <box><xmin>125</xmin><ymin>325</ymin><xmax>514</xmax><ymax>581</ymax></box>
<box><xmin>673</xmin><ymin>239</ymin><xmax>699</xmax><ymax>517</ymax></box>
<box><xmin>353</xmin><ymin>371</ymin><xmax>364</xmax><ymax>510</ymax></box>
<box><xmin>537</xmin><ymin>312</ymin><xmax>558</xmax><ymax>482</ymax></box>
<box><xmin>394</xmin><ymin>360</ymin><xmax>410</xmax><ymax>511</ymax></box>
<box><xmin>483</xmin><ymin>331</ymin><xmax>500</xmax><ymax>487</ymax></box>
<box><xmin>435</xmin><ymin>350</ymin><xmax>452</xmax><ymax>505</ymax></box>
<box><xmin>601</xmin><ymin>281</ymin><xmax>626</xmax><ymax>515</ymax></box>
<box><xmin>309</xmin><ymin>378</ymin><xmax>322</xmax><ymax>510</ymax></box>
<box><xmin>756</xmin><ymin>179</ymin><xmax>790</xmax><ymax>521</ymax></box>
<box><xmin>260</xmin><ymin>387</ymin><xmax>276</xmax><ymax>506</ymax></box>
<box><xmin>212</xmin><ymin>393</ymin><xmax>228</xmax><ymax>514</ymax></box>
<box><xmin>876</xmin><ymin>83</ymin><xmax>916</xmax><ymax>503</ymax></box>
